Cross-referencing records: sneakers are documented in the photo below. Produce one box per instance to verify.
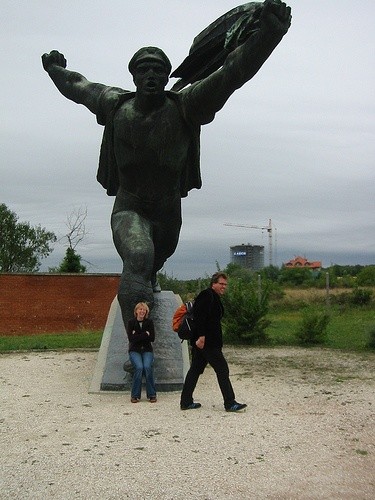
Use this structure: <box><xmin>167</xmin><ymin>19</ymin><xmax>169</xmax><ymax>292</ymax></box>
<box><xmin>226</xmin><ymin>403</ymin><xmax>247</xmax><ymax>411</ymax></box>
<box><xmin>181</xmin><ymin>403</ymin><xmax>201</xmax><ymax>410</ymax></box>
<box><xmin>131</xmin><ymin>398</ymin><xmax>137</xmax><ymax>402</ymax></box>
<box><xmin>150</xmin><ymin>397</ymin><xmax>156</xmax><ymax>402</ymax></box>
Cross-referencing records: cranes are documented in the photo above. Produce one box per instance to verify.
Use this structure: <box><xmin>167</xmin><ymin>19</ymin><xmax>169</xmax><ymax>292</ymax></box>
<box><xmin>223</xmin><ymin>218</ymin><xmax>278</xmax><ymax>266</ymax></box>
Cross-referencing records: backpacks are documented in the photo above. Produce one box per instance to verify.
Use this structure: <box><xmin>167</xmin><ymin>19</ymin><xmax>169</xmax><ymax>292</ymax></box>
<box><xmin>173</xmin><ymin>296</ymin><xmax>196</xmax><ymax>343</ymax></box>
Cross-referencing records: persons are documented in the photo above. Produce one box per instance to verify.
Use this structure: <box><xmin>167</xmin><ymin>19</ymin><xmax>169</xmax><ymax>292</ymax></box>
<box><xmin>40</xmin><ymin>0</ymin><xmax>293</xmax><ymax>374</ymax></box>
<box><xmin>128</xmin><ymin>303</ymin><xmax>157</xmax><ymax>403</ymax></box>
<box><xmin>180</xmin><ymin>273</ymin><xmax>247</xmax><ymax>412</ymax></box>
